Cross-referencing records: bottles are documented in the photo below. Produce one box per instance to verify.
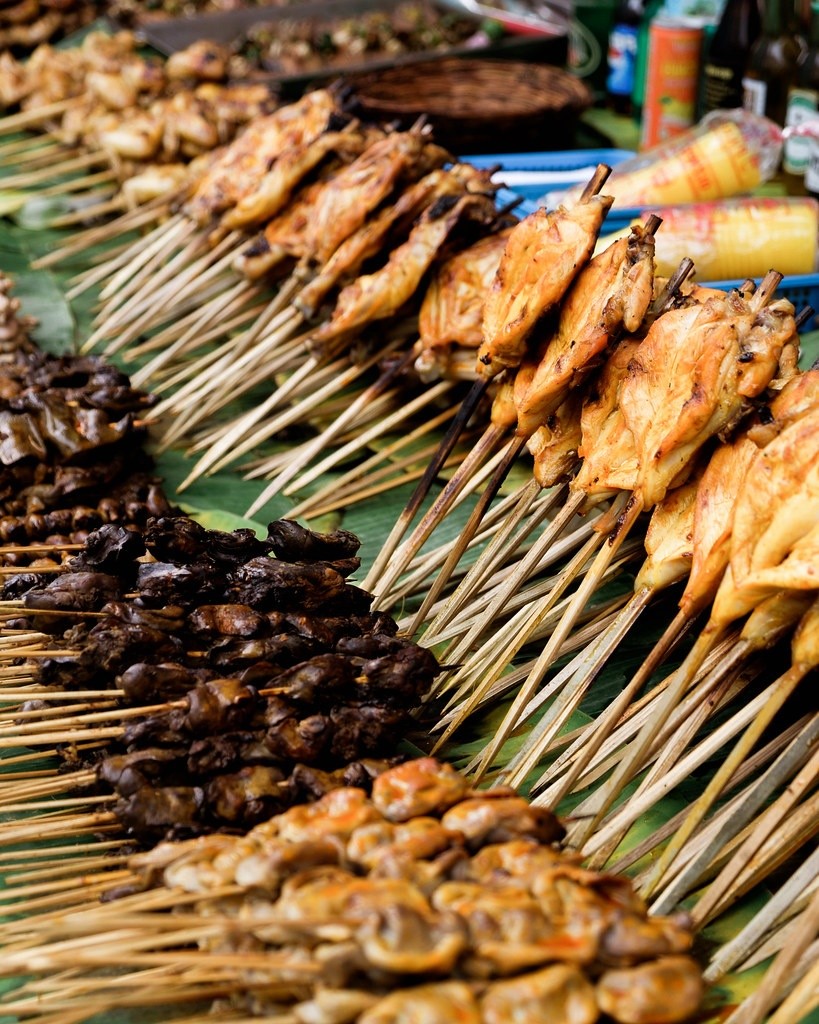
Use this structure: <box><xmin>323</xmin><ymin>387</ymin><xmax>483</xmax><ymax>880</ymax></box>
<box><xmin>604</xmin><ymin>0</ymin><xmax>819</xmax><ymax>200</ymax></box>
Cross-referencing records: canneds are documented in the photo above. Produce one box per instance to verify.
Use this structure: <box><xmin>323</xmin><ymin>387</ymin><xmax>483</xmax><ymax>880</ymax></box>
<box><xmin>640</xmin><ymin>14</ymin><xmax>703</xmax><ymax>150</ymax></box>
